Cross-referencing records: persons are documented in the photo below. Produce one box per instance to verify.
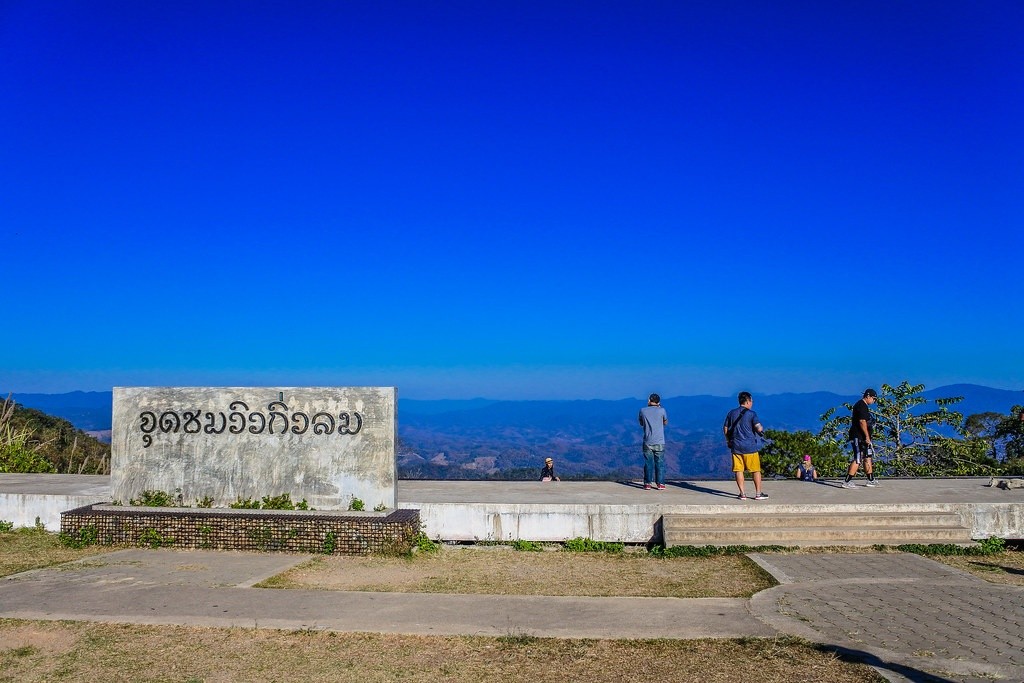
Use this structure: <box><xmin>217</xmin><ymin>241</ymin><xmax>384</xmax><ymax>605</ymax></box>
<box><xmin>539</xmin><ymin>458</ymin><xmax>561</xmax><ymax>482</ymax></box>
<box><xmin>840</xmin><ymin>388</ymin><xmax>882</xmax><ymax>490</ymax></box>
<box><xmin>722</xmin><ymin>391</ymin><xmax>770</xmax><ymax>499</ymax></box>
<box><xmin>638</xmin><ymin>393</ymin><xmax>670</xmax><ymax>489</ymax></box>
<box><xmin>796</xmin><ymin>455</ymin><xmax>817</xmax><ymax>482</ymax></box>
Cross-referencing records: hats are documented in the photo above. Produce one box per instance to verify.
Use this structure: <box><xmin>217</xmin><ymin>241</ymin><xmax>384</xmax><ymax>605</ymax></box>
<box><xmin>864</xmin><ymin>389</ymin><xmax>878</xmax><ymax>400</ymax></box>
<box><xmin>804</xmin><ymin>455</ymin><xmax>811</xmax><ymax>461</ymax></box>
<box><xmin>545</xmin><ymin>458</ymin><xmax>554</xmax><ymax>465</ymax></box>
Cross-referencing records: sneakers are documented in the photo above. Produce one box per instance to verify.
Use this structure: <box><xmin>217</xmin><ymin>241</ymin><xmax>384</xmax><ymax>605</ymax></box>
<box><xmin>756</xmin><ymin>493</ymin><xmax>769</xmax><ymax>500</ymax></box>
<box><xmin>865</xmin><ymin>479</ymin><xmax>885</xmax><ymax>487</ymax></box>
<box><xmin>643</xmin><ymin>483</ymin><xmax>652</xmax><ymax>489</ymax></box>
<box><xmin>842</xmin><ymin>481</ymin><xmax>856</xmax><ymax>488</ymax></box>
<box><xmin>657</xmin><ymin>483</ymin><xmax>666</xmax><ymax>489</ymax></box>
<box><xmin>738</xmin><ymin>494</ymin><xmax>747</xmax><ymax>500</ymax></box>
